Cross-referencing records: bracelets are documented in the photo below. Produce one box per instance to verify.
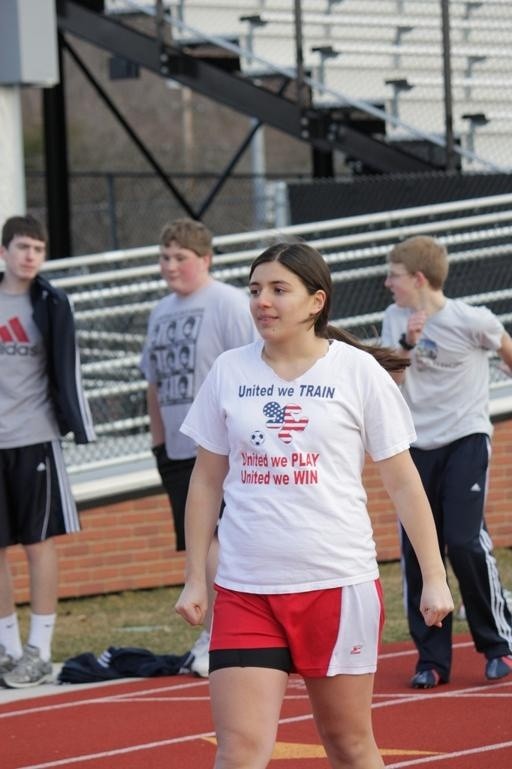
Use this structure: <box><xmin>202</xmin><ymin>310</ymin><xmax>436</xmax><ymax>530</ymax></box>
<box><xmin>398</xmin><ymin>333</ymin><xmax>416</xmax><ymax>351</ymax></box>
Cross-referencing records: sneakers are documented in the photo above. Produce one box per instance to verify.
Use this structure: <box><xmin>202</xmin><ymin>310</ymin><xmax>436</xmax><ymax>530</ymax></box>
<box><xmin>188</xmin><ymin>629</ymin><xmax>211</xmax><ymax>679</ymax></box>
<box><xmin>484</xmin><ymin>654</ymin><xmax>512</xmax><ymax>680</ymax></box>
<box><xmin>0</xmin><ymin>641</ymin><xmax>55</xmax><ymax>689</ymax></box>
<box><xmin>409</xmin><ymin>662</ymin><xmax>452</xmax><ymax>690</ymax></box>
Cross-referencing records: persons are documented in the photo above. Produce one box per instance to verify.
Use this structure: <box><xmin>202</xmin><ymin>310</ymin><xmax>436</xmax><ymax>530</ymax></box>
<box><xmin>172</xmin><ymin>245</ymin><xmax>455</xmax><ymax>769</ymax></box>
<box><xmin>136</xmin><ymin>215</ymin><xmax>265</xmax><ymax>679</ymax></box>
<box><xmin>376</xmin><ymin>233</ymin><xmax>512</xmax><ymax>688</ymax></box>
<box><xmin>0</xmin><ymin>214</ymin><xmax>97</xmax><ymax>688</ymax></box>
